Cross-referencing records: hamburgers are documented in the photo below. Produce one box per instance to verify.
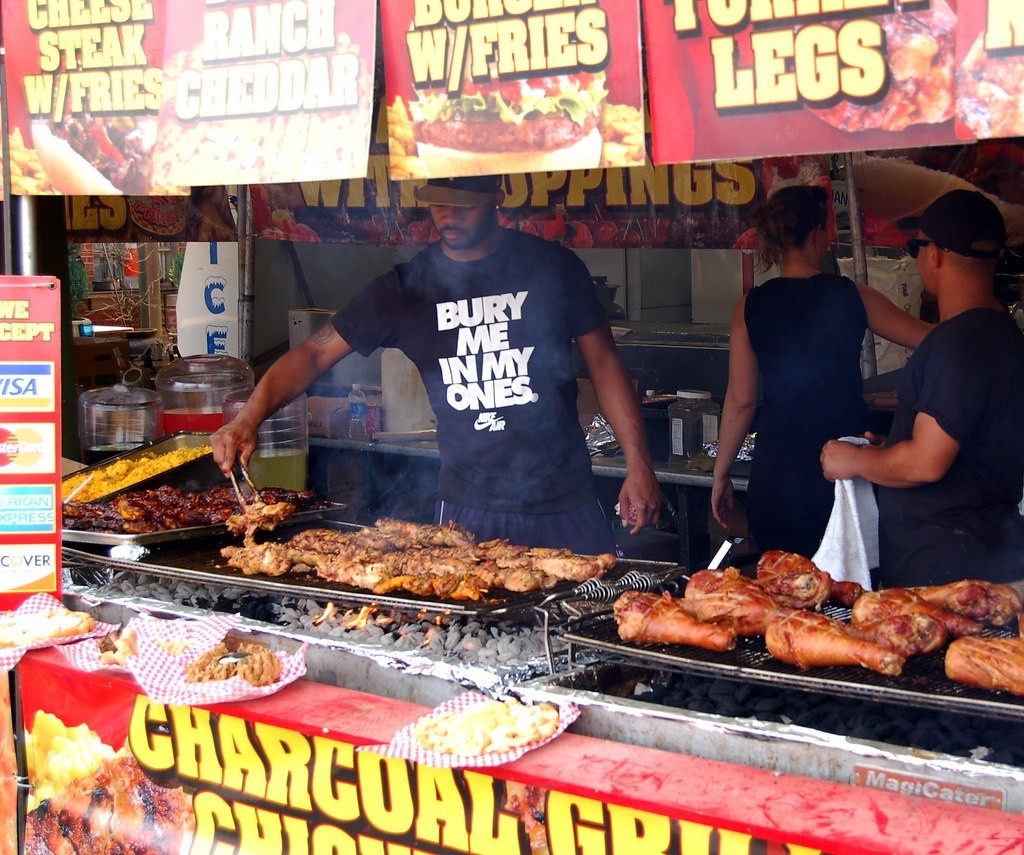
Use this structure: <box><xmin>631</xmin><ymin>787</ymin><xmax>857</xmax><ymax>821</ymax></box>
<box><xmin>410</xmin><ymin>22</ymin><xmax>602</xmax><ymax>177</ymax></box>
<box><xmin>32</xmin><ymin>115</ymin><xmax>156</xmax><ymax>195</ymax></box>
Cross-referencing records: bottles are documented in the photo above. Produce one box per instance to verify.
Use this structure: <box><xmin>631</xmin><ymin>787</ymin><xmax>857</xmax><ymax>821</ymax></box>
<box><xmin>348</xmin><ymin>384</ymin><xmax>367</xmax><ymax>440</ymax></box>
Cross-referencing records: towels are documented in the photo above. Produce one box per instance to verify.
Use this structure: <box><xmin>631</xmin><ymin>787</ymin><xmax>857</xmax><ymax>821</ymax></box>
<box><xmin>811</xmin><ymin>435</ymin><xmax>882</xmax><ymax>594</ymax></box>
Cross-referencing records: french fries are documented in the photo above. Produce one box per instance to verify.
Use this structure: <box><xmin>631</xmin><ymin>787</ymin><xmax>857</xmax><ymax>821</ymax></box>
<box><xmin>603</xmin><ymin>105</ymin><xmax>644</xmax><ymax>167</ymax></box>
<box><xmin>0</xmin><ymin>126</ymin><xmax>61</xmax><ymax>195</ymax></box>
<box><xmin>386</xmin><ymin>95</ymin><xmax>419</xmax><ymax>179</ymax></box>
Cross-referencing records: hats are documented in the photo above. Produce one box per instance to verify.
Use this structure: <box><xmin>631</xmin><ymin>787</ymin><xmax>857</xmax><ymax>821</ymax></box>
<box><xmin>414</xmin><ymin>175</ymin><xmax>503</xmax><ymax>206</ymax></box>
<box><xmin>896</xmin><ymin>189</ymin><xmax>1009</xmax><ymax>260</ymax></box>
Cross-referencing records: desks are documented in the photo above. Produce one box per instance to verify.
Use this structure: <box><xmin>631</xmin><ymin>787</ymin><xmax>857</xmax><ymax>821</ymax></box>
<box><xmin>74</xmin><ymin>338</ymin><xmax>130</xmax><ymax>387</ymax></box>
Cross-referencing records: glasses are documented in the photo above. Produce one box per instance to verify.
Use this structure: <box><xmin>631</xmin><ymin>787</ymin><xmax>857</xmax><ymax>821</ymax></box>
<box><xmin>906</xmin><ymin>240</ymin><xmax>950</xmax><ymax>259</ymax></box>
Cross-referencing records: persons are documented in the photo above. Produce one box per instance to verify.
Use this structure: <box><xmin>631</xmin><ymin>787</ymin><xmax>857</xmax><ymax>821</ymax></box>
<box><xmin>820</xmin><ymin>188</ymin><xmax>1024</xmax><ymax>610</ymax></box>
<box><xmin>209</xmin><ymin>173</ymin><xmax>663</xmax><ymax>555</ymax></box>
<box><xmin>708</xmin><ymin>184</ymin><xmax>940</xmax><ymax>593</ymax></box>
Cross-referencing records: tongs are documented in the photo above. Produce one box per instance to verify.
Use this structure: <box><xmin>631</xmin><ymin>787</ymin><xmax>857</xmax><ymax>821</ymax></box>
<box><xmin>227</xmin><ymin>467</ymin><xmax>263</xmax><ymax>511</ymax></box>
